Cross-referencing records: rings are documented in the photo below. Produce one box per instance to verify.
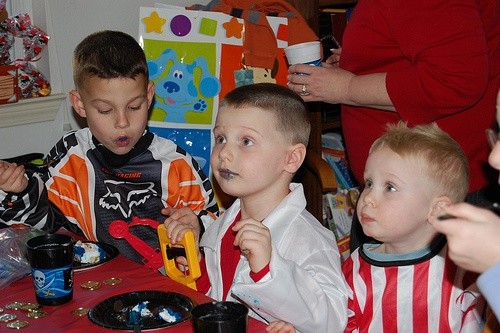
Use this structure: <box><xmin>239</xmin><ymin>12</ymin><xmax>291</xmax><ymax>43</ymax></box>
<box><xmin>302</xmin><ymin>85</ymin><xmax>307</xmax><ymax>95</ymax></box>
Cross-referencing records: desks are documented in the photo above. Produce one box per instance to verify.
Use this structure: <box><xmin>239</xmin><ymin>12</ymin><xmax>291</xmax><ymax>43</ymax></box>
<box><xmin>0</xmin><ymin>226</ymin><xmax>269</xmax><ymax>333</ymax></box>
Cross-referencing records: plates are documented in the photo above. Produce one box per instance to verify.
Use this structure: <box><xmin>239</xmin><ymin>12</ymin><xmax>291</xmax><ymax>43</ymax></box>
<box><xmin>88</xmin><ymin>290</ymin><xmax>199</xmax><ymax>331</ymax></box>
<box><xmin>72</xmin><ymin>241</ymin><xmax>120</xmax><ymax>272</ymax></box>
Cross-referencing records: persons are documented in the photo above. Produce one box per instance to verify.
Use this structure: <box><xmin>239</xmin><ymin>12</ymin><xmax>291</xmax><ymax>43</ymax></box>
<box><xmin>0</xmin><ymin>30</ymin><xmax>220</xmax><ymax>276</ymax></box>
<box><xmin>428</xmin><ymin>89</ymin><xmax>500</xmax><ymax>327</ymax></box>
<box><xmin>164</xmin><ymin>83</ymin><xmax>348</xmax><ymax>333</ymax></box>
<box><xmin>265</xmin><ymin>122</ymin><xmax>488</xmax><ymax>333</ymax></box>
<box><xmin>286</xmin><ymin>1</ymin><xmax>500</xmax><ymax>254</ymax></box>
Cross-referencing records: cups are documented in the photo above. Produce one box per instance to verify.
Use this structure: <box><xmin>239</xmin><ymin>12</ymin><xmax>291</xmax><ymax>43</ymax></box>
<box><xmin>284</xmin><ymin>40</ymin><xmax>323</xmax><ymax>76</ymax></box>
<box><xmin>26</xmin><ymin>234</ymin><xmax>75</xmax><ymax>306</ymax></box>
<box><xmin>317</xmin><ymin>35</ymin><xmax>342</xmax><ymax>68</ymax></box>
<box><xmin>190</xmin><ymin>301</ymin><xmax>249</xmax><ymax>333</ymax></box>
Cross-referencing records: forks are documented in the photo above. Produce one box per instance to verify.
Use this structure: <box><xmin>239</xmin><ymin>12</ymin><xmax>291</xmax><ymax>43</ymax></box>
<box><xmin>18</xmin><ymin>152</ymin><xmax>64</xmax><ymax>166</ymax></box>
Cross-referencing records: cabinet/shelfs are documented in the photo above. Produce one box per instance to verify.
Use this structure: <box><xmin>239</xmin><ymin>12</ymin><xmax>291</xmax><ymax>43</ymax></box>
<box><xmin>284</xmin><ymin>0</ymin><xmax>357</xmax><ymax>225</ymax></box>
